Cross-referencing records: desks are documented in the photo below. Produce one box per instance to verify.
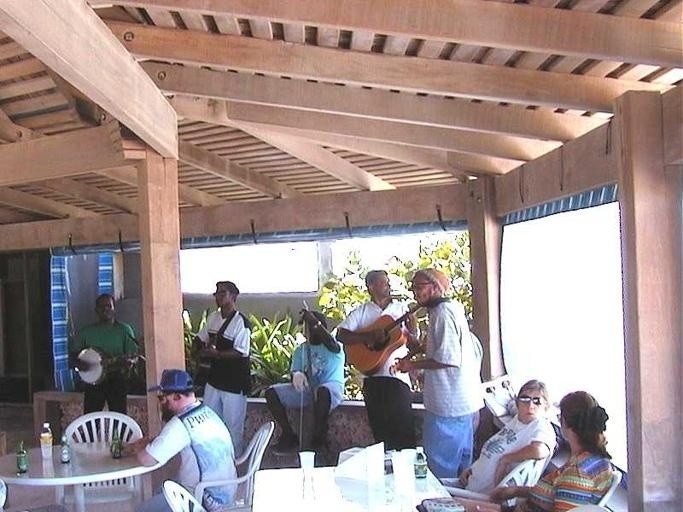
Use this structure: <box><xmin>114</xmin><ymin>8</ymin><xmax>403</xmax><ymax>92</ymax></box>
<box><xmin>253</xmin><ymin>465</ymin><xmax>457</xmax><ymax>511</ymax></box>
<box><xmin>0</xmin><ymin>442</ymin><xmax>162</xmax><ymax>512</ymax></box>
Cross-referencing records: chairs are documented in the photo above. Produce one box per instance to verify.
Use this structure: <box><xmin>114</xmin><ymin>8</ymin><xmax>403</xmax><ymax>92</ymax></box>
<box><xmin>439</xmin><ymin>442</ymin><xmax>560</xmax><ymax>508</ymax></box>
<box><xmin>192</xmin><ymin>419</ymin><xmax>277</xmax><ymax>511</ymax></box>
<box><xmin>595</xmin><ymin>468</ymin><xmax>624</xmax><ymax>511</ymax></box>
<box><xmin>54</xmin><ymin>410</ymin><xmax>143</xmax><ymax>511</ymax></box>
<box><xmin>162</xmin><ymin>479</ymin><xmax>209</xmax><ymax>511</ymax></box>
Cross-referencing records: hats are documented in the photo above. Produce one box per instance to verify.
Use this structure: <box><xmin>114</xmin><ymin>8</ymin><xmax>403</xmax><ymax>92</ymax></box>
<box><xmin>421</xmin><ymin>268</ymin><xmax>448</xmax><ymax>292</ymax></box>
<box><xmin>148</xmin><ymin>369</ymin><xmax>192</xmax><ymax>392</ymax></box>
<box><xmin>216</xmin><ymin>282</ymin><xmax>239</xmax><ymax>293</ymax></box>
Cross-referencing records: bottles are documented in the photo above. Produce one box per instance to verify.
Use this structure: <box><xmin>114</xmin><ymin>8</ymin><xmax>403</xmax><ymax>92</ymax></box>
<box><xmin>15</xmin><ymin>439</ymin><xmax>29</xmax><ymax>474</ymax></box>
<box><xmin>59</xmin><ymin>431</ymin><xmax>71</xmax><ymax>464</ymax></box>
<box><xmin>413</xmin><ymin>447</ymin><xmax>427</xmax><ymax>492</ymax></box>
<box><xmin>384</xmin><ymin>449</ymin><xmax>395</xmax><ymax>502</ymax></box>
<box><xmin>111</xmin><ymin>429</ymin><xmax>123</xmax><ymax>458</ymax></box>
<box><xmin>41</xmin><ymin>422</ymin><xmax>53</xmax><ymax>461</ymax></box>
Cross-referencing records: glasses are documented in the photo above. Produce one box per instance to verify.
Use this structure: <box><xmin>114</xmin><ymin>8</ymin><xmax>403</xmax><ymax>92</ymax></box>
<box><xmin>157</xmin><ymin>392</ymin><xmax>172</xmax><ymax>400</ymax></box>
<box><xmin>517</xmin><ymin>396</ymin><xmax>541</xmax><ymax>404</ymax></box>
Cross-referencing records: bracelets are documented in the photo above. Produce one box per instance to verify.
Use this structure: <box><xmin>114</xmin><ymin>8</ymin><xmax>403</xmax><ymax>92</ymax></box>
<box><xmin>497</xmin><ymin>458</ymin><xmax>504</xmax><ymax>470</ymax></box>
<box><xmin>312</xmin><ymin>321</ymin><xmax>321</xmax><ymax>330</ymax></box>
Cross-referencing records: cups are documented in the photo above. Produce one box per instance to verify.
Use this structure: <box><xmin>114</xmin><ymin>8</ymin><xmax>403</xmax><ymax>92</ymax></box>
<box><xmin>298</xmin><ymin>451</ymin><xmax>314</xmax><ymax>477</ymax></box>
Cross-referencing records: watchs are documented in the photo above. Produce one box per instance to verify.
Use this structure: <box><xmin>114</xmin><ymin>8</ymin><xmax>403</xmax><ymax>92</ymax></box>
<box><xmin>214</xmin><ymin>350</ymin><xmax>222</xmax><ymax>362</ymax></box>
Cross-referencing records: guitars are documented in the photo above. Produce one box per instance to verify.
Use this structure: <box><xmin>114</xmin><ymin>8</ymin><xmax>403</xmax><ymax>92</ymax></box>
<box><xmin>78</xmin><ymin>345</ymin><xmax>144</xmax><ymax>386</ymax></box>
<box><xmin>345</xmin><ymin>304</ymin><xmax>423</xmax><ymax>375</ymax></box>
<box><xmin>193</xmin><ymin>335</ymin><xmax>219</xmax><ymax>385</ymax></box>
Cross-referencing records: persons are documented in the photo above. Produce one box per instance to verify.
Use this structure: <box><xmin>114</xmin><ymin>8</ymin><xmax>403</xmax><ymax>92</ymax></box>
<box><xmin>122</xmin><ymin>367</ymin><xmax>240</xmax><ymax>512</ymax></box>
<box><xmin>440</xmin><ymin>380</ymin><xmax>559</xmax><ymax>507</ymax></box>
<box><xmin>189</xmin><ymin>280</ymin><xmax>252</xmax><ymax>460</ymax></box>
<box><xmin>393</xmin><ymin>268</ymin><xmax>488</xmax><ymax>482</ymax></box>
<box><xmin>452</xmin><ymin>390</ymin><xmax>614</xmax><ymax>512</ymax></box>
<box><xmin>265</xmin><ymin>308</ymin><xmax>345</xmax><ymax>457</ymax></box>
<box><xmin>469</xmin><ymin>330</ymin><xmax>484</xmax><ymax>435</ymax></box>
<box><xmin>68</xmin><ymin>293</ymin><xmax>140</xmax><ymax>445</ymax></box>
<box><xmin>335</xmin><ymin>270</ymin><xmax>422</xmax><ymax>451</ymax></box>
